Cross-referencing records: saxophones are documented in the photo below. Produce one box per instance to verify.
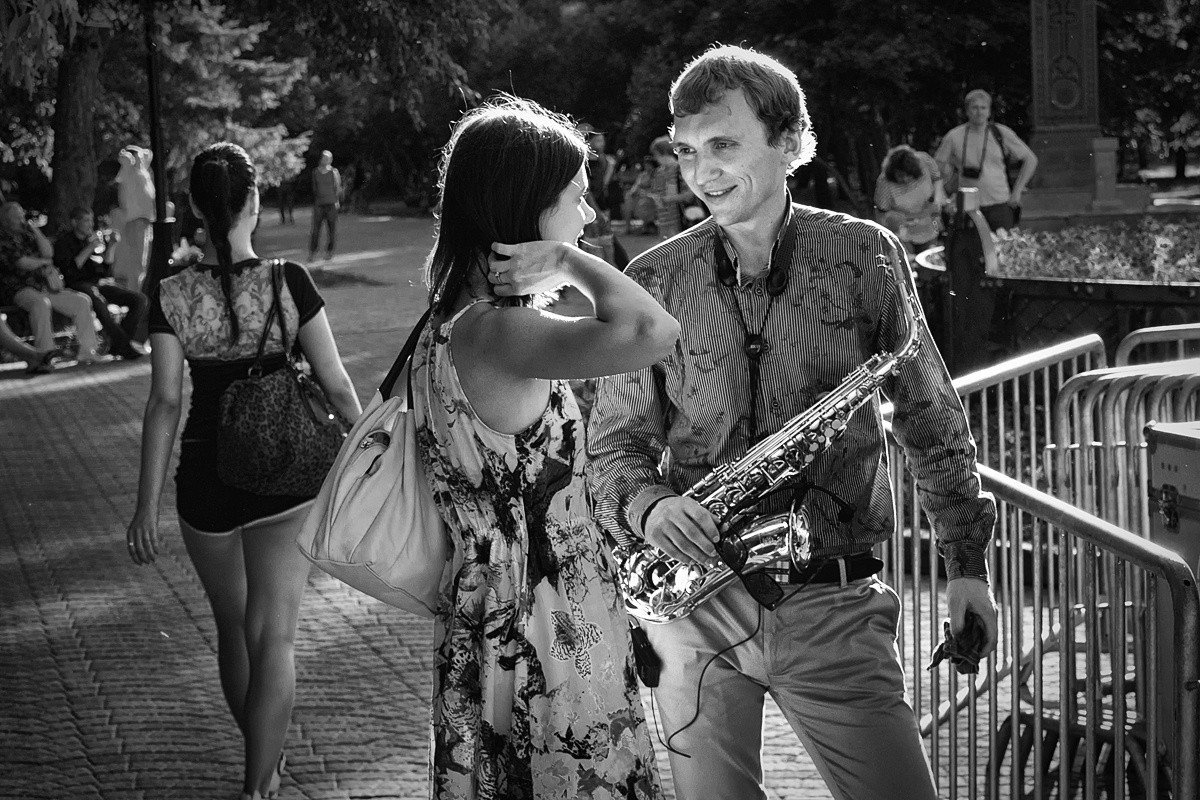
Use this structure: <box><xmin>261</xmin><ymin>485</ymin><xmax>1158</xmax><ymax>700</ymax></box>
<box><xmin>618</xmin><ymin>229</ymin><xmax>923</xmax><ymax>625</ymax></box>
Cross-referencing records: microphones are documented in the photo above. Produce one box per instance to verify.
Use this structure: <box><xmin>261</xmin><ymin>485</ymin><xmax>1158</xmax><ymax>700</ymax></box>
<box><xmin>963</xmin><ymin>167</ymin><xmax>981</xmax><ymax>178</ymax></box>
<box><xmin>803</xmin><ymin>483</ymin><xmax>855</xmax><ymax>523</ymax></box>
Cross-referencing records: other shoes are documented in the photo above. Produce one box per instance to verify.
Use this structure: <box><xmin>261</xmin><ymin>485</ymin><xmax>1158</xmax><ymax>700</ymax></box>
<box><xmin>86</xmin><ymin>355</ymin><xmax>114</xmax><ymax>365</ymax></box>
<box><xmin>109</xmin><ymin>344</ymin><xmax>143</xmax><ymax>358</ymax></box>
<box><xmin>26</xmin><ymin>349</ymin><xmax>64</xmax><ymax>374</ymax></box>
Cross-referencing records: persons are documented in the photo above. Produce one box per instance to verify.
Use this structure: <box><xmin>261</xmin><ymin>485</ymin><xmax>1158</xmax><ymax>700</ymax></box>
<box><xmin>580</xmin><ymin>131</ymin><xmax>694</xmax><ymax>268</ymax></box>
<box><xmin>586</xmin><ymin>43</ymin><xmax>998</xmax><ymax>800</ymax></box>
<box><xmin>278</xmin><ymin>181</ymin><xmax>296</xmax><ymax>224</ymax></box>
<box><xmin>875</xmin><ymin>90</ymin><xmax>1038</xmax><ymax>255</ymax></box>
<box><xmin>410</xmin><ymin>91</ymin><xmax>682</xmax><ymax>800</ymax></box>
<box><xmin>0</xmin><ymin>145</ymin><xmax>189</xmax><ymax>376</ymax></box>
<box><xmin>307</xmin><ymin>150</ymin><xmax>341</xmax><ymax>262</ymax></box>
<box><xmin>127</xmin><ymin>143</ymin><xmax>363</xmax><ymax>800</ymax></box>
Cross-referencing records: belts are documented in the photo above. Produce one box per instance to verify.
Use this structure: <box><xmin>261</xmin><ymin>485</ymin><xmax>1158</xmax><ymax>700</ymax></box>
<box><xmin>764</xmin><ymin>548</ymin><xmax>884</xmax><ymax>584</ymax></box>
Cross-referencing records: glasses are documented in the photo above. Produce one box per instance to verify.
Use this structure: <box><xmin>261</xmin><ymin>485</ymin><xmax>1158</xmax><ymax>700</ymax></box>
<box><xmin>713</xmin><ymin>482</ymin><xmax>857</xmax><ymax>611</ymax></box>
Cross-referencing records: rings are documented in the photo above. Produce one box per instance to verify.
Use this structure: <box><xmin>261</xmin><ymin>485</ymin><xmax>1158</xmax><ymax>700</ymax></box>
<box><xmin>129</xmin><ymin>542</ymin><xmax>134</xmax><ymax>547</ymax></box>
<box><xmin>494</xmin><ymin>271</ymin><xmax>504</xmax><ymax>285</ymax></box>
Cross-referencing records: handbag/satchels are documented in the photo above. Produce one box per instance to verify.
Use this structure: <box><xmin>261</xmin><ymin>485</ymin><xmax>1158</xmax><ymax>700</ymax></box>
<box><xmin>294</xmin><ymin>306</ymin><xmax>445</xmax><ymax>619</ymax></box>
<box><xmin>45</xmin><ymin>265</ymin><xmax>64</xmax><ymax>292</ymax></box>
<box><xmin>1007</xmin><ymin>177</ymin><xmax>1021</xmax><ymax>224</ymax></box>
<box><xmin>212</xmin><ymin>258</ymin><xmax>354</xmax><ymax>500</ymax></box>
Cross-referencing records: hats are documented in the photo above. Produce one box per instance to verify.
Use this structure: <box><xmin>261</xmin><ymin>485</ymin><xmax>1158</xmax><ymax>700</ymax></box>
<box><xmin>585</xmin><ymin>131</ymin><xmax>609</xmax><ymax>140</ymax></box>
